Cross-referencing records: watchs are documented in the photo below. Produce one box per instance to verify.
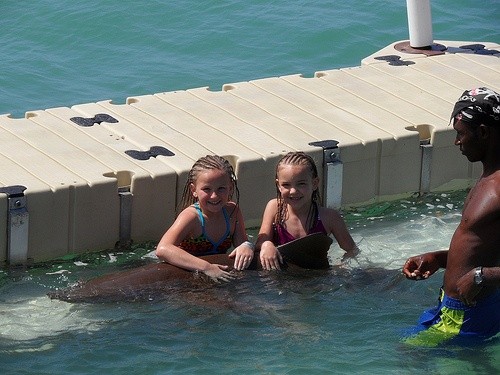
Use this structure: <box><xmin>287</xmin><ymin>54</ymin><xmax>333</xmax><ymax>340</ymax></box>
<box><xmin>473</xmin><ymin>267</ymin><xmax>482</xmax><ymax>284</ymax></box>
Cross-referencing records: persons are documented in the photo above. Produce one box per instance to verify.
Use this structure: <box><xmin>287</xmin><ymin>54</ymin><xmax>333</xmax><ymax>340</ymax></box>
<box><xmin>254</xmin><ymin>152</ymin><xmax>359</xmax><ymax>271</ymax></box>
<box><xmin>156</xmin><ymin>155</ymin><xmax>254</xmax><ymax>284</ymax></box>
<box><xmin>403</xmin><ymin>88</ymin><xmax>500</xmax><ymax>349</ymax></box>
